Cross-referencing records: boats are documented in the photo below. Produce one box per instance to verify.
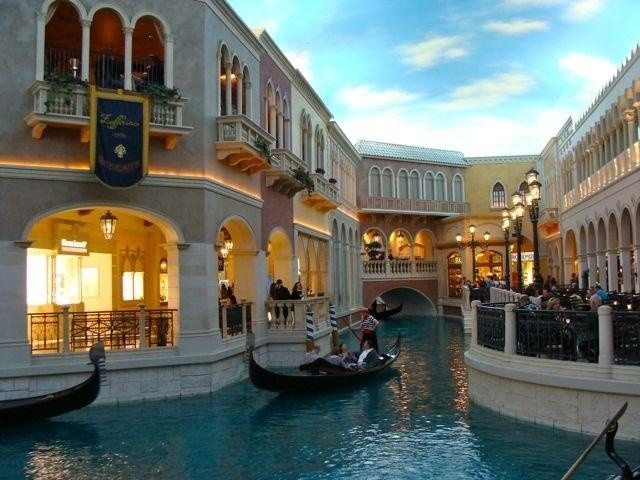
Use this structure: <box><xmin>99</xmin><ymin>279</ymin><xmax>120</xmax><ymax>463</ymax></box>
<box><xmin>0</xmin><ymin>340</ymin><xmax>106</xmax><ymax>426</ymax></box>
<box><xmin>368</xmin><ymin>300</ymin><xmax>404</xmax><ymax>321</ymax></box>
<box><xmin>247</xmin><ymin>332</ymin><xmax>402</xmax><ymax>394</ymax></box>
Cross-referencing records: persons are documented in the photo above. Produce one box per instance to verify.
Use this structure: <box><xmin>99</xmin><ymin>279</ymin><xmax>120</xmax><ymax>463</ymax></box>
<box><xmin>358</xmin><ymin>307</ymin><xmax>381</xmax><ymax>355</ymax></box>
<box><xmin>348</xmin><ymin>340</ymin><xmax>379</xmax><ymax>371</ymax></box>
<box><xmin>388</xmin><ymin>254</ymin><xmax>394</xmax><ymax>260</ymax></box>
<box><xmin>218</xmin><ymin>278</ymin><xmax>306</xmax><ymax>337</ymax></box>
<box><xmin>328</xmin><ymin>347</ymin><xmax>344</xmax><ymax>366</ymax></box>
<box><xmin>450</xmin><ymin>268</ymin><xmax>620</xmax><ymax>365</ymax></box>
<box><xmin>378</xmin><ymin>253</ymin><xmax>385</xmax><ymax>260</ymax></box>
<box><xmin>368</xmin><ymin>255</ymin><xmax>378</xmax><ymax>260</ymax></box>
<box><xmin>338</xmin><ymin>342</ymin><xmax>358</xmax><ymax>362</ymax></box>
<box><xmin>91</xmin><ymin>48</ymin><xmax>166</xmax><ymax>95</ymax></box>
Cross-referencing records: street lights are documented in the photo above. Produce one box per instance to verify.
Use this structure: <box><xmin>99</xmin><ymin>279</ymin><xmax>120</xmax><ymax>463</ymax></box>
<box><xmin>525</xmin><ymin>166</ymin><xmax>542</xmax><ymax>293</ymax></box>
<box><xmin>501</xmin><ymin>201</ymin><xmax>513</xmax><ymax>295</ymax></box>
<box><xmin>456</xmin><ymin>223</ymin><xmax>490</xmax><ymax>281</ymax></box>
<box><xmin>510</xmin><ymin>188</ymin><xmax>530</xmax><ymax>294</ymax></box>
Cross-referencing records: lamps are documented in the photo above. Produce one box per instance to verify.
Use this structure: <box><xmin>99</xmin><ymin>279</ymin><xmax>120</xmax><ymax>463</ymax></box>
<box><xmin>373</xmin><ymin>230</ymin><xmax>381</xmax><ymax>243</ymax></box>
<box><xmin>99</xmin><ymin>209</ymin><xmax>119</xmax><ymax>240</ymax></box>
<box><xmin>220</xmin><ymin>234</ymin><xmax>233</xmax><ymax>258</ymax></box>
<box><xmin>397</xmin><ymin>231</ymin><xmax>405</xmax><ymax>243</ymax></box>
